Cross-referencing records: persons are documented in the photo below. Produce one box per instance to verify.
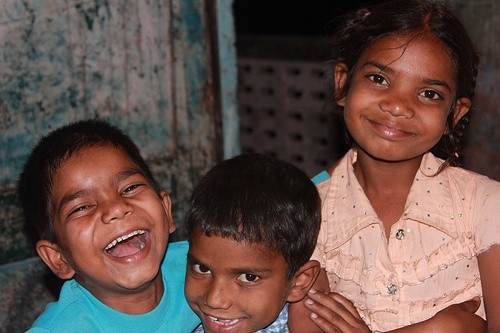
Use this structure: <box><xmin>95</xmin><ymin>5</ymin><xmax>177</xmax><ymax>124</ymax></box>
<box><xmin>18</xmin><ymin>120</ymin><xmax>333</xmax><ymax>333</ymax></box>
<box><xmin>304</xmin><ymin>1</ymin><xmax>500</xmax><ymax>333</ymax></box>
<box><xmin>182</xmin><ymin>153</ymin><xmax>374</xmax><ymax>333</ymax></box>
